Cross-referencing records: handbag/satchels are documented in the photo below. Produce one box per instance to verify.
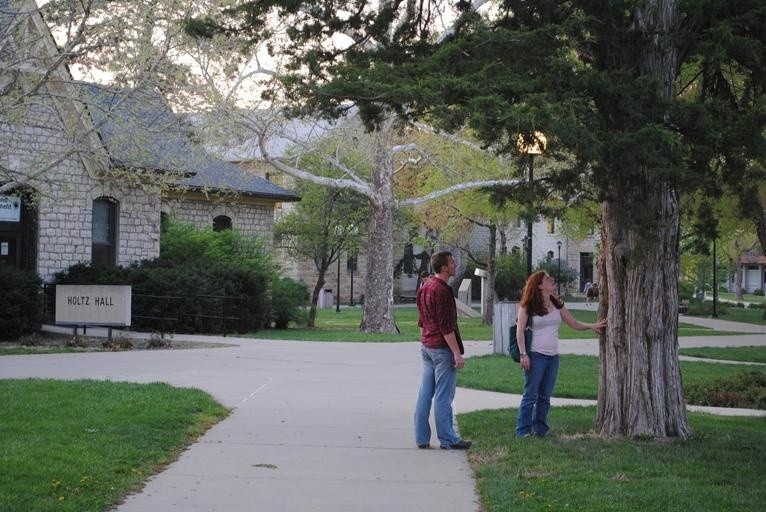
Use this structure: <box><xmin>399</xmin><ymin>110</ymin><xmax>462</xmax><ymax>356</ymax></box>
<box><xmin>509</xmin><ymin>325</ymin><xmax>532</xmax><ymax>362</ymax></box>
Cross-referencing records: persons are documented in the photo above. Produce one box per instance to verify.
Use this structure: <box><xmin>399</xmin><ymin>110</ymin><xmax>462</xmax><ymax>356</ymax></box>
<box><xmin>418</xmin><ymin>272</ymin><xmax>431</xmax><ymax>289</ymax></box>
<box><xmin>515</xmin><ymin>270</ymin><xmax>607</xmax><ymax>437</ymax></box>
<box><xmin>414</xmin><ymin>251</ymin><xmax>472</xmax><ymax>448</ymax></box>
<box><xmin>583</xmin><ymin>279</ymin><xmax>593</xmax><ymax>306</ymax></box>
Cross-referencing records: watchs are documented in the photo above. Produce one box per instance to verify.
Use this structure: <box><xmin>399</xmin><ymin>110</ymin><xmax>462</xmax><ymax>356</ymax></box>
<box><xmin>519</xmin><ymin>352</ymin><xmax>527</xmax><ymax>356</ymax></box>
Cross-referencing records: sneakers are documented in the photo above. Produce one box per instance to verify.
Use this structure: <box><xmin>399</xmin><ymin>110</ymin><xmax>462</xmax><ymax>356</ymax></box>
<box><xmin>440</xmin><ymin>440</ymin><xmax>472</xmax><ymax>449</ymax></box>
<box><xmin>418</xmin><ymin>444</ymin><xmax>429</xmax><ymax>448</ymax></box>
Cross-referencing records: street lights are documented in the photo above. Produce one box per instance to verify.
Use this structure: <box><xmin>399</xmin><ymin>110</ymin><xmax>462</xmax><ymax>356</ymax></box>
<box><xmin>515</xmin><ymin>130</ymin><xmax>548</xmax><ymax>286</ymax></box>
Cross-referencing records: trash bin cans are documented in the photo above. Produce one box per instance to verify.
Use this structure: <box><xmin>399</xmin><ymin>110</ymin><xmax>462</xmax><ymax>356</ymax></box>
<box><xmin>317</xmin><ymin>288</ymin><xmax>333</xmax><ymax>310</ymax></box>
<box><xmin>494</xmin><ymin>301</ymin><xmax>522</xmax><ymax>355</ymax></box>
<box><xmin>696</xmin><ymin>288</ymin><xmax>706</xmax><ymax>303</ymax></box>
